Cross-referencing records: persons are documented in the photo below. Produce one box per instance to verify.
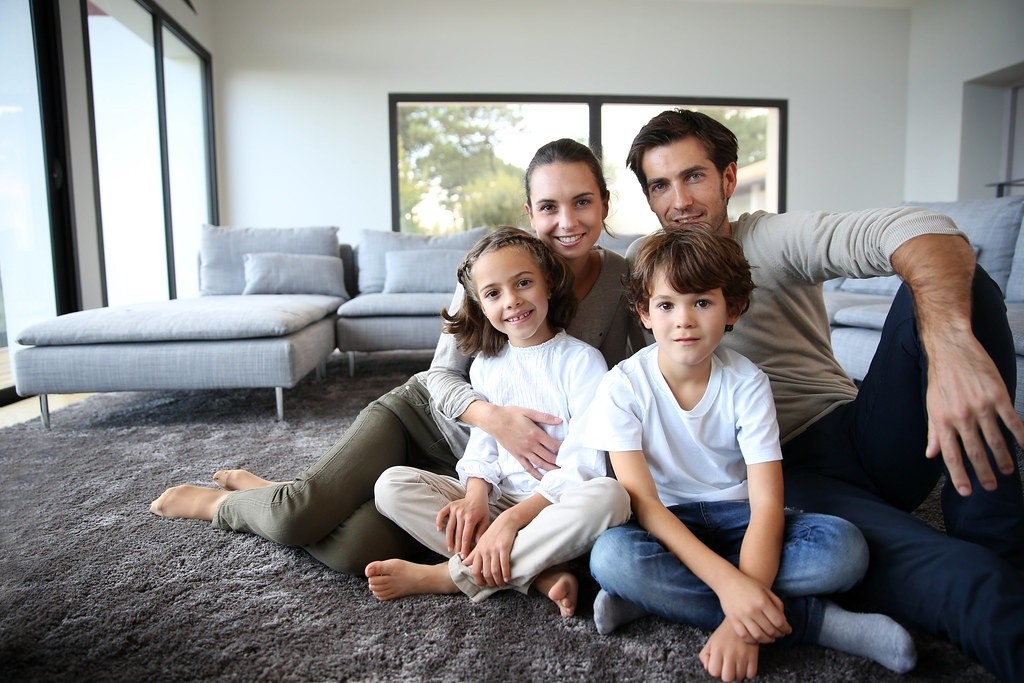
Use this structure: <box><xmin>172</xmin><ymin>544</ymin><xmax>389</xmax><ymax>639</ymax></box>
<box><xmin>149</xmin><ymin>137</ymin><xmax>633</xmax><ymax>571</ymax></box>
<box><xmin>583</xmin><ymin>222</ymin><xmax>914</xmax><ymax>683</ymax></box>
<box><xmin>624</xmin><ymin>109</ymin><xmax>1024</xmax><ymax>682</ymax></box>
<box><xmin>366</xmin><ymin>227</ymin><xmax>631</xmax><ymax>617</ymax></box>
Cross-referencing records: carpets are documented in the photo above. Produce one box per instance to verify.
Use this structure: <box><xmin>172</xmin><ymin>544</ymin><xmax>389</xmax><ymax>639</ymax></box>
<box><xmin>0</xmin><ymin>349</ymin><xmax>1024</xmax><ymax>683</ymax></box>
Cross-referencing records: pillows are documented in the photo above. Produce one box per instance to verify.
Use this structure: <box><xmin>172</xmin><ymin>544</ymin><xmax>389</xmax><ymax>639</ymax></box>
<box><xmin>382</xmin><ymin>250</ymin><xmax>467</xmax><ymax>294</ymax></box>
<box><xmin>239</xmin><ymin>251</ymin><xmax>352</xmax><ymax>300</ymax></box>
<box><xmin>839</xmin><ymin>194</ymin><xmax>1024</xmax><ymax>303</ymax></box>
<box><xmin>199</xmin><ymin>223</ymin><xmax>340</xmax><ymax>296</ymax></box>
<box><xmin>357</xmin><ymin>225</ymin><xmax>490</xmax><ymax>294</ymax></box>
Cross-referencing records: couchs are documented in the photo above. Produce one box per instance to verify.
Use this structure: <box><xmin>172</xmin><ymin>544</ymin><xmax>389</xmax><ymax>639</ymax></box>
<box><xmin>13</xmin><ymin>223</ymin><xmax>358</xmax><ymax>430</ymax></box>
<box><xmin>336</xmin><ymin>194</ymin><xmax>1024</xmax><ymax>524</ymax></box>
